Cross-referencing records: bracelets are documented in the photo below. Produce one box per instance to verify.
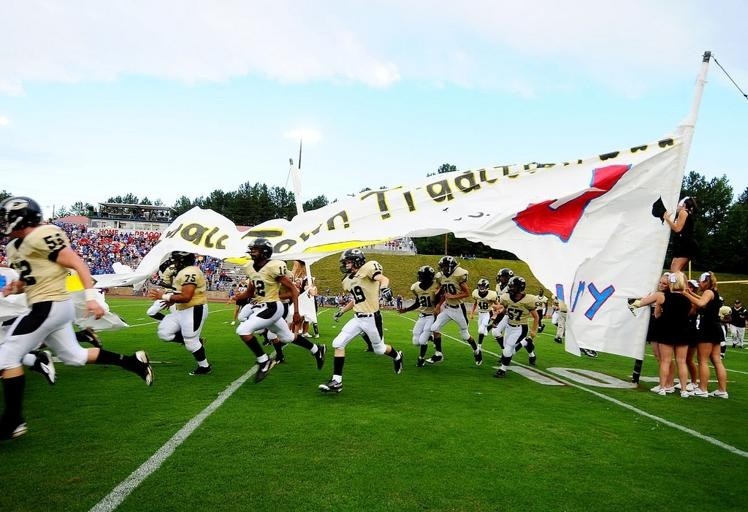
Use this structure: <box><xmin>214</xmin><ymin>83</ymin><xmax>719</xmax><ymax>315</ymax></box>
<box><xmin>162</xmin><ymin>294</ymin><xmax>172</xmax><ymax>302</ymax></box>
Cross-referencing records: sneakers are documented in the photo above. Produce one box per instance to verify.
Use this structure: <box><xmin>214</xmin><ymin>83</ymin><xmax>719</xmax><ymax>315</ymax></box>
<box><xmin>732</xmin><ymin>344</ymin><xmax>744</xmax><ymax>349</ymax></box>
<box><xmin>1</xmin><ymin>418</ymin><xmax>27</xmax><ymax>439</ymax></box>
<box><xmin>319</xmin><ymin>380</ymin><xmax>343</xmax><ymax>393</ymax></box>
<box><xmin>650</xmin><ymin>382</ymin><xmax>729</xmax><ymax>399</ymax></box>
<box><xmin>392</xmin><ymin>351</ymin><xmax>403</xmax><ymax>375</ymax></box>
<box><xmin>537</xmin><ymin>324</ymin><xmax>545</xmax><ymax>333</ymax></box>
<box><xmin>315</xmin><ymin>344</ymin><xmax>327</xmax><ymax>369</ymax></box>
<box><xmin>188</xmin><ymin>366</ymin><xmax>212</xmax><ymax>376</ymax></box>
<box><xmin>35</xmin><ymin>327</ymin><xmax>103</xmax><ymax>385</ymax></box>
<box><xmin>255</xmin><ymin>359</ymin><xmax>277</xmax><ymax>383</ymax></box>
<box><xmin>580</xmin><ymin>347</ymin><xmax>599</xmax><ymax>359</ymax></box>
<box><xmin>554</xmin><ymin>337</ymin><xmax>562</xmax><ymax>343</ymax></box>
<box><xmin>412</xmin><ymin>332</ymin><xmax>537</xmax><ymax>379</ymax></box>
<box><xmin>199</xmin><ymin>338</ymin><xmax>204</xmax><ymax>350</ymax></box>
<box><xmin>273</xmin><ymin>357</ymin><xmax>285</xmax><ymax>367</ymax></box>
<box><xmin>720</xmin><ymin>353</ymin><xmax>725</xmax><ymax>360</ymax></box>
<box><xmin>262</xmin><ymin>329</ymin><xmax>320</xmax><ymax>346</ymax></box>
<box><xmin>133</xmin><ymin>349</ymin><xmax>154</xmax><ymax>387</ymax></box>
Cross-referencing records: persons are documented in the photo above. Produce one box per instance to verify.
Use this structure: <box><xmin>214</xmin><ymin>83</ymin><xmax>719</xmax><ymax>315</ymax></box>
<box><xmin>1</xmin><ymin>237</ymin><xmax>10</xmax><ymax>266</ymax></box>
<box><xmin>144</xmin><ymin>260</ymin><xmax>177</xmax><ymax>321</ymax></box>
<box><xmin>149</xmin><ymin>252</ymin><xmax>215</xmax><ymax>379</ymax></box>
<box><xmin>397</xmin><ymin>264</ymin><xmax>441</xmax><ymax>370</ymax></box>
<box><xmin>51</xmin><ymin>220</ymin><xmax>161</xmax><ymax>275</ymax></box>
<box><xmin>316</xmin><ymin>248</ymin><xmax>405</xmax><ymax>395</ymax></box>
<box><xmin>318</xmin><ymin>289</ymin><xmax>354</xmax><ymax>307</ymax></box>
<box><xmin>1</xmin><ymin>266</ymin><xmax>103</xmax><ymax>388</ymax></box>
<box><xmin>0</xmin><ymin>195</ymin><xmax>155</xmax><ymax>442</ymax></box>
<box><xmin>197</xmin><ymin>255</ymin><xmax>248</xmax><ymax>297</ymax></box>
<box><xmin>229</xmin><ymin>237</ymin><xmax>328</xmax><ymax>383</ymax></box>
<box><xmin>468</xmin><ymin>267</ymin><xmax>567</xmax><ymax>379</ymax></box>
<box><xmin>425</xmin><ymin>255</ymin><xmax>483</xmax><ymax>367</ymax></box>
<box><xmin>627</xmin><ymin>271</ymin><xmax>747</xmax><ymax>402</ymax></box>
<box><xmin>388</xmin><ymin>239</ymin><xmax>414</xmax><ymax>249</ymax></box>
<box><xmin>663</xmin><ymin>196</ymin><xmax>699</xmax><ymax>292</ymax></box>
<box><xmin>384</xmin><ymin>295</ymin><xmax>403</xmax><ymax>310</ymax></box>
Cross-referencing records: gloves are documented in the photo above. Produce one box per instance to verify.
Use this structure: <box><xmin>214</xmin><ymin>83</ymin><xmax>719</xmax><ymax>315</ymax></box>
<box><xmin>380</xmin><ymin>288</ymin><xmax>393</xmax><ymax>302</ymax></box>
<box><xmin>333</xmin><ymin>311</ymin><xmax>343</xmax><ymax>322</ymax></box>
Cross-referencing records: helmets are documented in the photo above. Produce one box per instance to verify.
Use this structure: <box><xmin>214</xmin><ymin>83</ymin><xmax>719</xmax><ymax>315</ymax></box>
<box><xmin>0</xmin><ymin>196</ymin><xmax>42</xmax><ymax>238</ymax></box>
<box><xmin>340</xmin><ymin>248</ymin><xmax>365</xmax><ymax>270</ymax></box>
<box><xmin>417</xmin><ymin>256</ymin><xmax>525</xmax><ymax>296</ymax></box>
<box><xmin>172</xmin><ymin>250</ymin><xmax>196</xmax><ymax>268</ymax></box>
<box><xmin>159</xmin><ymin>260</ymin><xmax>174</xmax><ymax>271</ymax></box>
<box><xmin>249</xmin><ymin>239</ymin><xmax>273</xmax><ymax>259</ymax></box>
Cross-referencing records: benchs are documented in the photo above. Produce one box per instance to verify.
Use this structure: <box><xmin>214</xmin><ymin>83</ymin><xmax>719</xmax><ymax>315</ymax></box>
<box><xmin>56</xmin><ymin>223</ymin><xmax>252</xmax><ymax>294</ymax></box>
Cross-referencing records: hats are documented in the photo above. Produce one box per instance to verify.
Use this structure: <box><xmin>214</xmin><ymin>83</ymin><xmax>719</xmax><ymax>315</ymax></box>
<box><xmin>735</xmin><ymin>300</ymin><xmax>741</xmax><ymax>304</ymax></box>
